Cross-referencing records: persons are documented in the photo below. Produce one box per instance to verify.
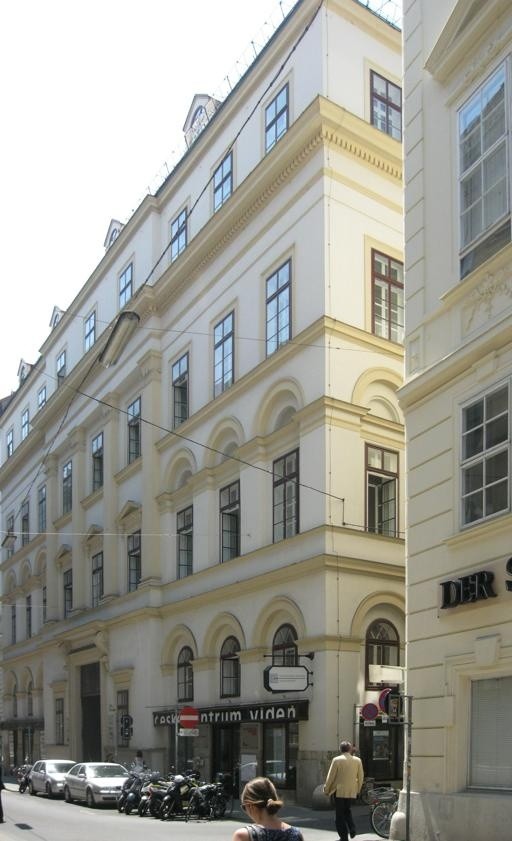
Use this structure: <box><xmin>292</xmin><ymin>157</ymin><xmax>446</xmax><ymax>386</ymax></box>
<box><xmin>321</xmin><ymin>739</ymin><xmax>365</xmax><ymax>841</ymax></box>
<box><xmin>233</xmin><ymin>776</ymin><xmax>305</xmax><ymax>841</ymax></box>
<box><xmin>106</xmin><ymin>753</ymin><xmax>114</xmax><ymax>763</ymax></box>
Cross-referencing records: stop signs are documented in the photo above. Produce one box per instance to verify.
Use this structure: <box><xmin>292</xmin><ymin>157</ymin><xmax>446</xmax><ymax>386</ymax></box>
<box><xmin>179</xmin><ymin>706</ymin><xmax>199</xmax><ymax>728</ymax></box>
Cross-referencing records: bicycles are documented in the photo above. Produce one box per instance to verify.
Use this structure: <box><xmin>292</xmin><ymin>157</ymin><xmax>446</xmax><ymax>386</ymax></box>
<box><xmin>362</xmin><ymin>776</ymin><xmax>400</xmax><ymax>839</ymax></box>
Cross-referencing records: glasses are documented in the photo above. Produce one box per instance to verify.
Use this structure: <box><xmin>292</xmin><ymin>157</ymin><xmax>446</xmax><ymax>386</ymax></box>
<box><xmin>240</xmin><ymin>800</ymin><xmax>254</xmax><ymax>814</ymax></box>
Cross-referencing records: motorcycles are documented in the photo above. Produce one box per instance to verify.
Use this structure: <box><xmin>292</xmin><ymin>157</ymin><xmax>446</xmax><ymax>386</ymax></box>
<box><xmin>17</xmin><ymin>765</ymin><xmax>30</xmax><ymax>795</ymax></box>
<box><xmin>119</xmin><ymin>766</ymin><xmax>235</xmax><ymax>822</ymax></box>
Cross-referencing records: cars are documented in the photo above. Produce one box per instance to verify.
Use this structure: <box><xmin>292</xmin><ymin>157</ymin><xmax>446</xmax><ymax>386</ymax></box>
<box><xmin>26</xmin><ymin>759</ymin><xmax>129</xmax><ymax>808</ymax></box>
<box><xmin>239</xmin><ymin>760</ymin><xmax>287</xmax><ymax>794</ymax></box>
